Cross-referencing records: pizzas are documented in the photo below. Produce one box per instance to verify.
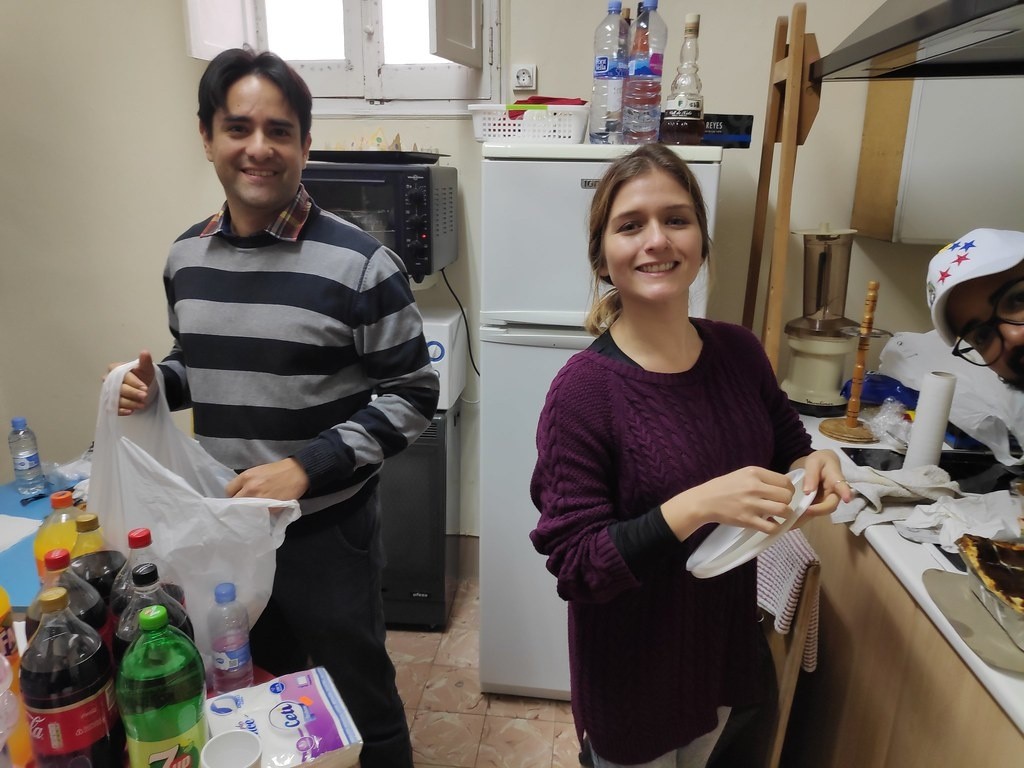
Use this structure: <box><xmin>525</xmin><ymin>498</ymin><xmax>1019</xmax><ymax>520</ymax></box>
<box><xmin>962</xmin><ymin>534</ymin><xmax>1024</xmax><ymax>616</ymax></box>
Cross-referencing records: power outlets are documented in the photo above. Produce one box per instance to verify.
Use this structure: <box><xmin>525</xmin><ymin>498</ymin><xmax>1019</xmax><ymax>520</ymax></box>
<box><xmin>511</xmin><ymin>64</ymin><xmax>536</xmax><ymax>90</ymax></box>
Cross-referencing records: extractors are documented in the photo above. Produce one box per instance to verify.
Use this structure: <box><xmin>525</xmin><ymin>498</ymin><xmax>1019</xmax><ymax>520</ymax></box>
<box><xmin>808</xmin><ymin>0</ymin><xmax>1024</xmax><ymax>83</ymax></box>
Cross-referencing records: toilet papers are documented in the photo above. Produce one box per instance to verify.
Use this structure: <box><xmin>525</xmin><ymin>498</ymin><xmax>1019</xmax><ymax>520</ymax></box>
<box><xmin>902</xmin><ymin>370</ymin><xmax>958</xmax><ymax>471</ymax></box>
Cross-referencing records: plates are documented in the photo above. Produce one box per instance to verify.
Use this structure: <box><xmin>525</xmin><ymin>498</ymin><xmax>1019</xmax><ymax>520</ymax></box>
<box><xmin>306</xmin><ymin>149</ymin><xmax>451</xmax><ymax>164</ymax></box>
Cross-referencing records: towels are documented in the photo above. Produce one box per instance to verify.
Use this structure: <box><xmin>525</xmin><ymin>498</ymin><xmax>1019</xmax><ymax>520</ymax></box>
<box><xmin>757</xmin><ymin>526</ymin><xmax>822</xmax><ymax>673</ymax></box>
<box><xmin>829</xmin><ymin>463</ymin><xmax>972</xmax><ymax>537</ymax></box>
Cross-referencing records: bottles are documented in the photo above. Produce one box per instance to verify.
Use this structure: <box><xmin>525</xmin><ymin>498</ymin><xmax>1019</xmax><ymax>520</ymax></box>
<box><xmin>0</xmin><ymin>490</ymin><xmax>209</xmax><ymax>768</ymax></box>
<box><xmin>8</xmin><ymin>416</ymin><xmax>44</xmax><ymax>494</ymax></box>
<box><xmin>589</xmin><ymin>0</ymin><xmax>705</xmax><ymax>144</ymax></box>
<box><xmin>208</xmin><ymin>583</ymin><xmax>254</xmax><ymax>697</ymax></box>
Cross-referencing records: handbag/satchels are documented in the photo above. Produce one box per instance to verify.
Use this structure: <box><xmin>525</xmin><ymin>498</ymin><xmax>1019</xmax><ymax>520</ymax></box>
<box><xmin>87</xmin><ymin>358</ymin><xmax>303</xmax><ymax>688</ymax></box>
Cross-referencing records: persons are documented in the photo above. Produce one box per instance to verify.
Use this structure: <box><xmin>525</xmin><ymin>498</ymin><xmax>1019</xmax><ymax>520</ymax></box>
<box><xmin>103</xmin><ymin>48</ymin><xmax>440</xmax><ymax>768</ymax></box>
<box><xmin>530</xmin><ymin>146</ymin><xmax>851</xmax><ymax>768</ymax></box>
<box><xmin>927</xmin><ymin>227</ymin><xmax>1024</xmax><ymax>391</ymax></box>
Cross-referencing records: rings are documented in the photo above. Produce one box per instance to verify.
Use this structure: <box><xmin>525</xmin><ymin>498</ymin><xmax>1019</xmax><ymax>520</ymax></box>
<box><xmin>835</xmin><ymin>479</ymin><xmax>851</xmax><ymax>488</ymax></box>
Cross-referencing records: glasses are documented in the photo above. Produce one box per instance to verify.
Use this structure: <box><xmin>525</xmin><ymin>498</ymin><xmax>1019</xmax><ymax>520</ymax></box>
<box><xmin>950</xmin><ymin>277</ymin><xmax>1024</xmax><ymax>367</ymax></box>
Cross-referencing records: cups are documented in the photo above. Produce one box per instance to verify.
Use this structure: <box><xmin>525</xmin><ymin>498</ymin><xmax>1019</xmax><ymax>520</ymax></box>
<box><xmin>201</xmin><ymin>729</ymin><xmax>263</xmax><ymax>768</ymax></box>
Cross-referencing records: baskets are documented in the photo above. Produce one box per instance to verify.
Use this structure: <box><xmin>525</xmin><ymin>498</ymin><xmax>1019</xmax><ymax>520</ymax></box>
<box><xmin>469</xmin><ymin>103</ymin><xmax>589</xmax><ymax>145</ymax></box>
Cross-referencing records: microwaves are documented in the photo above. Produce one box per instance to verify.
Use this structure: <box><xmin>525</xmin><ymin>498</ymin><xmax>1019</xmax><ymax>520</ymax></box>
<box><xmin>298</xmin><ymin>160</ymin><xmax>462</xmax><ymax>285</ymax></box>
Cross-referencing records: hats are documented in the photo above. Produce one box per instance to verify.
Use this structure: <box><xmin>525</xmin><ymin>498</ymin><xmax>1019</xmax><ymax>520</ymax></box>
<box><xmin>925</xmin><ymin>229</ymin><xmax>1024</xmax><ymax>346</ymax></box>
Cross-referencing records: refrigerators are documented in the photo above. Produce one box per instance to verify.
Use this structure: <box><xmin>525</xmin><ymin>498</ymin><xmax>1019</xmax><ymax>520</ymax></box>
<box><xmin>479</xmin><ymin>140</ymin><xmax>728</xmax><ymax>697</ymax></box>
